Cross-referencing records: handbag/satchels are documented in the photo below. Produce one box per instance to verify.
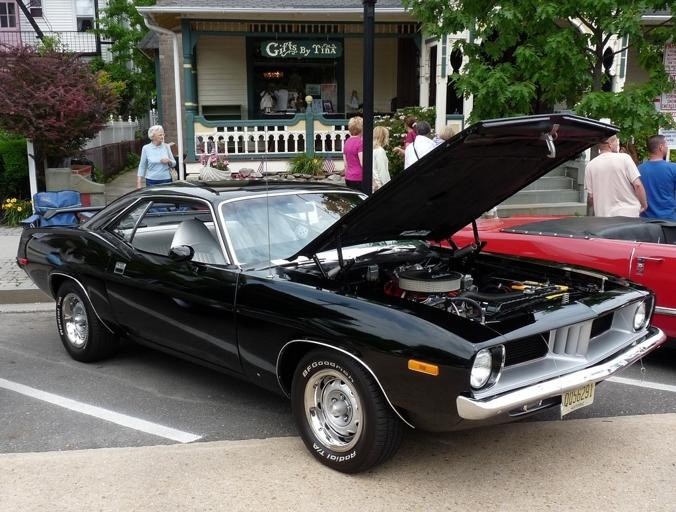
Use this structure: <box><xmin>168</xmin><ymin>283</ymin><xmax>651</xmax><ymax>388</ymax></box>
<box><xmin>169</xmin><ymin>167</ymin><xmax>179</xmax><ymax>182</ymax></box>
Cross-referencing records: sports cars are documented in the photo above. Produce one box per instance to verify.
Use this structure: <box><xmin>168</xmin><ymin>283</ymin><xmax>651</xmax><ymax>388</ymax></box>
<box><xmin>432</xmin><ymin>212</ymin><xmax>676</xmax><ymax>345</ymax></box>
<box><xmin>12</xmin><ymin>111</ymin><xmax>668</xmax><ymax>473</ymax></box>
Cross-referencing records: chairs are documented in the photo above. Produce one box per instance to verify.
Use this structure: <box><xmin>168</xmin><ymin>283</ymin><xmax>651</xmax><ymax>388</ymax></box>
<box><xmin>170</xmin><ymin>219</ymin><xmax>228</xmax><ymax>265</ymax></box>
<box><xmin>19</xmin><ymin>190</ymin><xmax>95</xmax><ymax>228</ymax></box>
<box><xmin>246</xmin><ymin>204</ymin><xmax>299</xmax><ymax>246</ymax></box>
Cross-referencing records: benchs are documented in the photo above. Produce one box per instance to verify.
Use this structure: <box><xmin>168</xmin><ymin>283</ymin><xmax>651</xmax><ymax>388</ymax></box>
<box><xmin>116</xmin><ymin>219</ymin><xmax>254</xmax><ymax>260</ymax></box>
<box><xmin>598</xmin><ymin>222</ymin><xmax>669</xmax><ymax>245</ymax></box>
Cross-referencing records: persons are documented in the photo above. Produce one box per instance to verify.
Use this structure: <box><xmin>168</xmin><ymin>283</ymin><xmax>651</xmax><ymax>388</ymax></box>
<box><xmin>350</xmin><ymin>90</ymin><xmax>359</xmax><ymax>111</ymax></box>
<box><xmin>371</xmin><ymin>126</ymin><xmax>391</xmax><ymax>194</ymax></box>
<box><xmin>343</xmin><ymin>115</ymin><xmax>363</xmax><ymax>192</ymax></box>
<box><xmin>433</xmin><ymin>127</ymin><xmax>454</xmax><ymax>145</ymax></box>
<box><xmin>404</xmin><ymin>118</ymin><xmax>417</xmax><ymax>147</ymax></box>
<box><xmin>636</xmin><ymin>135</ymin><xmax>676</xmax><ymax>222</ymax></box>
<box><xmin>137</xmin><ymin>124</ymin><xmax>177</xmax><ymax>189</ymax></box>
<box><xmin>404</xmin><ymin>121</ymin><xmax>436</xmax><ymax>169</ymax></box>
<box><xmin>584</xmin><ymin>134</ymin><xmax>648</xmax><ymax>218</ymax></box>
<box><xmin>260</xmin><ymin>87</ymin><xmax>274</xmax><ymax>113</ymax></box>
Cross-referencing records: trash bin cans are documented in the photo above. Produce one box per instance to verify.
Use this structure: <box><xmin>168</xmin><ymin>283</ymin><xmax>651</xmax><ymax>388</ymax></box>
<box><xmin>70</xmin><ymin>165</ymin><xmax>92</xmax><ymax>181</ymax></box>
